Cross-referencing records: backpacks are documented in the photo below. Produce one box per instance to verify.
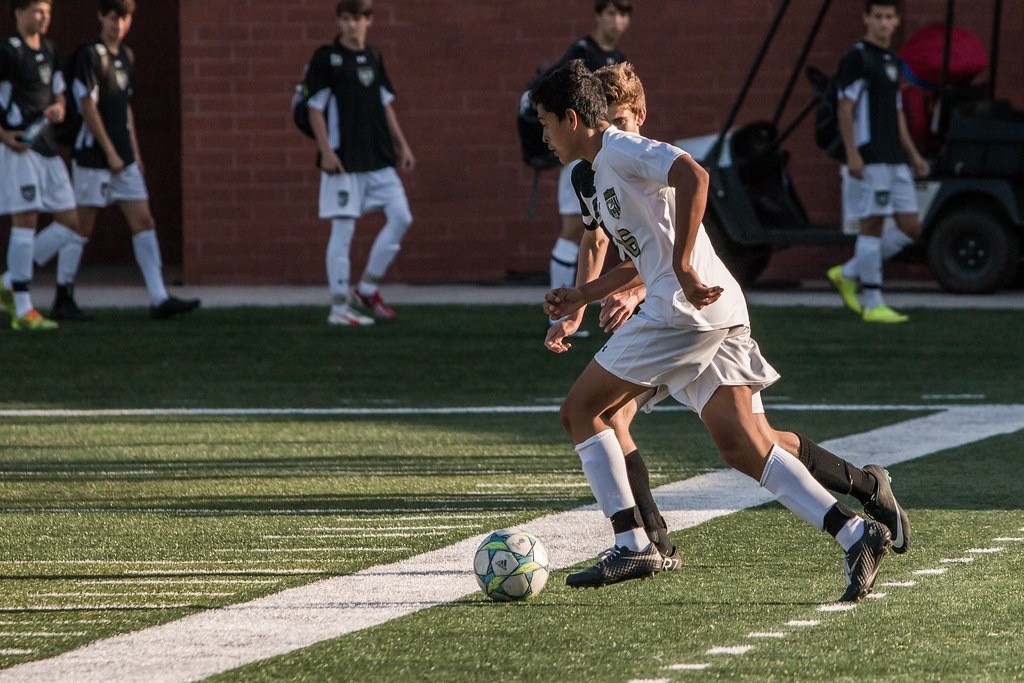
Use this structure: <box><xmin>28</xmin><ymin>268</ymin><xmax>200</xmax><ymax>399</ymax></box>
<box><xmin>517</xmin><ymin>91</ymin><xmax>557</xmax><ymax>169</ymax></box>
<box><xmin>292</xmin><ymin>47</ymin><xmax>378</xmax><ymax>140</ymax></box>
<box><xmin>813</xmin><ymin>41</ymin><xmax>865</xmax><ymax>165</ymax></box>
<box><xmin>54</xmin><ymin>38</ymin><xmax>135</xmax><ymax>147</ymax></box>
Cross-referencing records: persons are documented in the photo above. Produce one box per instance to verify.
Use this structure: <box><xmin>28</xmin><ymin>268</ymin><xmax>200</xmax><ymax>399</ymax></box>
<box><xmin>0</xmin><ymin>0</ymin><xmax>77</xmax><ymax>328</ymax></box>
<box><xmin>550</xmin><ymin>0</ymin><xmax>632</xmax><ymax>337</ymax></box>
<box><xmin>525</xmin><ymin>58</ymin><xmax>910</xmax><ymax>602</ymax></box>
<box><xmin>52</xmin><ymin>0</ymin><xmax>201</xmax><ymax>322</ymax></box>
<box><xmin>303</xmin><ymin>0</ymin><xmax>414</xmax><ymax>326</ymax></box>
<box><xmin>828</xmin><ymin>0</ymin><xmax>930</xmax><ymax>323</ymax></box>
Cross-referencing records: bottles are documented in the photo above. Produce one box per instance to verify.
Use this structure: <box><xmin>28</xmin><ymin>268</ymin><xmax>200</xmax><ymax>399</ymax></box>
<box><xmin>23</xmin><ymin>116</ymin><xmax>50</xmax><ymax>147</ymax></box>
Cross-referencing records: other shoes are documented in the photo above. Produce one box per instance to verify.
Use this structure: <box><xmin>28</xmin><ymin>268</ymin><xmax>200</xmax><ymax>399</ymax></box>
<box><xmin>148</xmin><ymin>295</ymin><xmax>201</xmax><ymax>318</ymax></box>
<box><xmin>49</xmin><ymin>300</ymin><xmax>97</xmax><ymax>323</ymax></box>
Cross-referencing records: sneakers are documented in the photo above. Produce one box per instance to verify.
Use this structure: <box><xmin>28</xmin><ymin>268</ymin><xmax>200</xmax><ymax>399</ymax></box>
<box><xmin>660</xmin><ymin>545</ymin><xmax>683</xmax><ymax>571</ymax></box>
<box><xmin>857</xmin><ymin>465</ymin><xmax>910</xmax><ymax>553</ymax></box>
<box><xmin>861</xmin><ymin>304</ymin><xmax>909</xmax><ymax>324</ymax></box>
<box><xmin>840</xmin><ymin>519</ymin><xmax>890</xmax><ymax>602</ymax></box>
<box><xmin>565</xmin><ymin>542</ymin><xmax>664</xmax><ymax>588</ymax></box>
<box><xmin>353</xmin><ymin>287</ymin><xmax>396</xmax><ymax>320</ymax></box>
<box><xmin>828</xmin><ymin>266</ymin><xmax>864</xmax><ymax>314</ymax></box>
<box><xmin>11</xmin><ymin>309</ymin><xmax>59</xmax><ymax>332</ymax></box>
<box><xmin>326</xmin><ymin>305</ymin><xmax>373</xmax><ymax>326</ymax></box>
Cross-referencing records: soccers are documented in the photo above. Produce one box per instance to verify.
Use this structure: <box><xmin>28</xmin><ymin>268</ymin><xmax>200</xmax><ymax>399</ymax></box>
<box><xmin>472</xmin><ymin>527</ymin><xmax>551</xmax><ymax>602</ymax></box>
